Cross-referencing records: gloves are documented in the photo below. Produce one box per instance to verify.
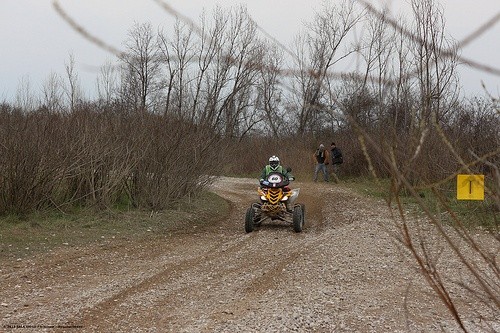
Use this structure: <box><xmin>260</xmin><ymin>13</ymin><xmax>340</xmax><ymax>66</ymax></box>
<box><xmin>260</xmin><ymin>179</ymin><xmax>264</xmax><ymax>181</ymax></box>
<box><xmin>289</xmin><ymin>178</ymin><xmax>294</xmax><ymax>181</ymax></box>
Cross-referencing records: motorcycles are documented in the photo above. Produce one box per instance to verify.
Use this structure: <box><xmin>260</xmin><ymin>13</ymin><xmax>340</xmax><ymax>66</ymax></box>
<box><xmin>243</xmin><ymin>168</ymin><xmax>304</xmax><ymax>232</ymax></box>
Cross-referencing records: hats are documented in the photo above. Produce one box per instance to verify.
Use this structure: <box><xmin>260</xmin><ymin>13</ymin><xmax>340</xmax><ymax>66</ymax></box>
<box><xmin>320</xmin><ymin>144</ymin><xmax>325</xmax><ymax>149</ymax></box>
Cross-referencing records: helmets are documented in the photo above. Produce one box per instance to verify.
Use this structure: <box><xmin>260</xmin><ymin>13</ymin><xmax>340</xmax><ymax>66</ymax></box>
<box><xmin>269</xmin><ymin>156</ymin><xmax>280</xmax><ymax>171</ymax></box>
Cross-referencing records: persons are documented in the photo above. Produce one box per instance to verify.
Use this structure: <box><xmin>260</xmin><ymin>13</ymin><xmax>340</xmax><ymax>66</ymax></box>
<box><xmin>313</xmin><ymin>144</ymin><xmax>330</xmax><ymax>183</ymax></box>
<box><xmin>331</xmin><ymin>142</ymin><xmax>343</xmax><ymax>183</ymax></box>
<box><xmin>259</xmin><ymin>155</ymin><xmax>295</xmax><ymax>211</ymax></box>
